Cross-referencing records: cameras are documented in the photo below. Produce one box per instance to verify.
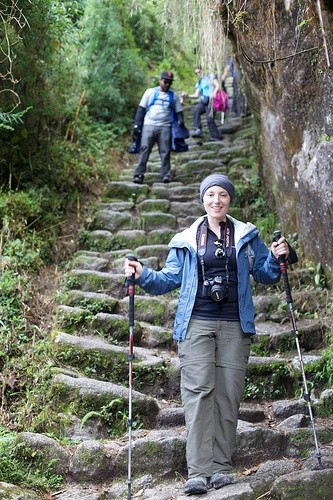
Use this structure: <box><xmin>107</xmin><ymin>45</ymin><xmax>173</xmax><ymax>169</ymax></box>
<box><xmin>202</xmin><ymin>276</ymin><xmax>230</xmax><ymax>302</ymax></box>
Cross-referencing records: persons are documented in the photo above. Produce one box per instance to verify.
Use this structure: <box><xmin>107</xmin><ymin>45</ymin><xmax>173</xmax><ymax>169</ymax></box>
<box><xmin>181</xmin><ymin>66</ymin><xmax>222</xmax><ymax>141</ymax></box>
<box><xmin>122</xmin><ymin>172</ymin><xmax>291</xmax><ymax>496</ymax></box>
<box><xmin>220</xmin><ymin>47</ymin><xmax>250</xmax><ymax>118</ymax></box>
<box><xmin>128</xmin><ymin>70</ymin><xmax>185</xmax><ymax>183</ymax></box>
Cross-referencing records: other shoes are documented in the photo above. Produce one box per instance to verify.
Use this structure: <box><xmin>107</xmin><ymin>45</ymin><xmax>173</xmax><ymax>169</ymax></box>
<box><xmin>163</xmin><ymin>176</ymin><xmax>170</xmax><ymax>183</ymax></box>
<box><xmin>231</xmin><ymin>112</ymin><xmax>238</xmax><ymax>117</ymax></box>
<box><xmin>135</xmin><ymin>176</ymin><xmax>143</xmax><ymax>183</ymax></box>
<box><xmin>192</xmin><ymin>132</ymin><xmax>204</xmax><ymax>137</ymax></box>
<box><xmin>241</xmin><ymin>112</ymin><xmax>247</xmax><ymax>117</ymax></box>
<box><xmin>210</xmin><ymin>473</ymin><xmax>236</xmax><ymax>488</ymax></box>
<box><xmin>210</xmin><ymin>137</ymin><xmax>222</xmax><ymax>141</ymax></box>
<box><xmin>184</xmin><ymin>477</ymin><xmax>208</xmax><ymax>493</ymax></box>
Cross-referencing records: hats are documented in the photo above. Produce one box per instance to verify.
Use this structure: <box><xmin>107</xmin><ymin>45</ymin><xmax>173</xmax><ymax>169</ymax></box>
<box><xmin>161</xmin><ymin>71</ymin><xmax>174</xmax><ymax>81</ymax></box>
<box><xmin>200</xmin><ymin>174</ymin><xmax>235</xmax><ymax>203</ymax></box>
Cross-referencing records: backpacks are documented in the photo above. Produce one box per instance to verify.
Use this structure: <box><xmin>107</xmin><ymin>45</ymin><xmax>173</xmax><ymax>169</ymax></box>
<box><xmin>214</xmin><ymin>89</ymin><xmax>230</xmax><ymax>111</ymax></box>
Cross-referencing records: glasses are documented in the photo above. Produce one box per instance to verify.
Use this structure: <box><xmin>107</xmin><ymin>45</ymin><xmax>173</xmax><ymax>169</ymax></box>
<box><xmin>163</xmin><ymin>80</ymin><xmax>172</xmax><ymax>85</ymax></box>
<box><xmin>213</xmin><ymin>239</ymin><xmax>226</xmax><ymax>258</ymax></box>
